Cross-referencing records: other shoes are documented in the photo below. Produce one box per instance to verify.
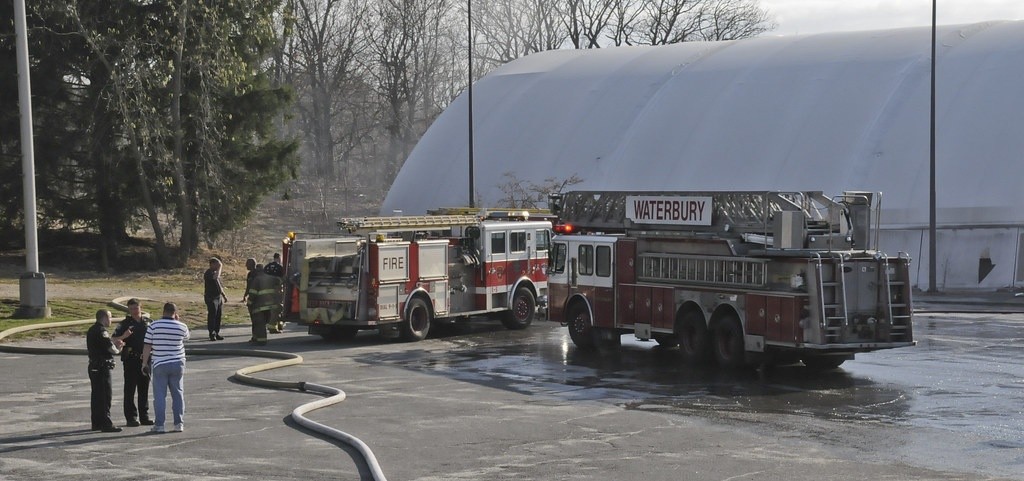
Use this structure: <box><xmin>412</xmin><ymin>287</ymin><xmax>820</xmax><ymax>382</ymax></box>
<box><xmin>151</xmin><ymin>425</ymin><xmax>164</xmax><ymax>433</ymax></box>
<box><xmin>270</xmin><ymin>329</ymin><xmax>281</xmax><ymax>334</ymax></box>
<box><xmin>91</xmin><ymin>422</ymin><xmax>102</xmax><ymax>431</ymax></box>
<box><xmin>102</xmin><ymin>425</ymin><xmax>122</xmax><ymax>432</ymax></box>
<box><xmin>216</xmin><ymin>335</ymin><xmax>224</xmax><ymax>340</ymax></box>
<box><xmin>141</xmin><ymin>420</ymin><xmax>153</xmax><ymax>425</ymax></box>
<box><xmin>174</xmin><ymin>423</ymin><xmax>183</xmax><ymax>432</ymax></box>
<box><xmin>209</xmin><ymin>334</ymin><xmax>216</xmax><ymax>341</ymax></box>
<box><xmin>248</xmin><ymin>337</ymin><xmax>267</xmax><ymax>346</ymax></box>
<box><xmin>127</xmin><ymin>419</ymin><xmax>139</xmax><ymax>426</ymax></box>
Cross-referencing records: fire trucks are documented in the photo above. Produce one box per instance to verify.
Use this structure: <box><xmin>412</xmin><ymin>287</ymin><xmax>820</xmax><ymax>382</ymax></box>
<box><xmin>280</xmin><ymin>207</ymin><xmax>556</xmax><ymax>344</ymax></box>
<box><xmin>548</xmin><ymin>188</ymin><xmax>918</xmax><ymax>377</ymax></box>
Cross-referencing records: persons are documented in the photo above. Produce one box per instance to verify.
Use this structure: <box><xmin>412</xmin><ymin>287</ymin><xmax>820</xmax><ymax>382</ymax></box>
<box><xmin>204</xmin><ymin>257</ymin><xmax>228</xmax><ymax>341</ymax></box>
<box><xmin>87</xmin><ymin>297</ymin><xmax>190</xmax><ymax>433</ymax></box>
<box><xmin>242</xmin><ymin>253</ymin><xmax>286</xmax><ymax>346</ymax></box>
